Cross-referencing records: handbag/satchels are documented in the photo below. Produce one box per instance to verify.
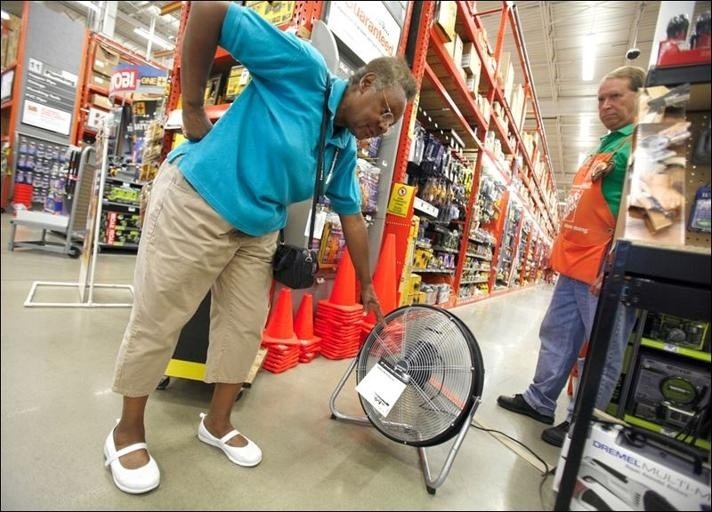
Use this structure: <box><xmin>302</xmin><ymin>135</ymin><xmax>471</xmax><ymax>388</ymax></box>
<box><xmin>269</xmin><ymin>242</ymin><xmax>320</xmax><ymax>292</ymax></box>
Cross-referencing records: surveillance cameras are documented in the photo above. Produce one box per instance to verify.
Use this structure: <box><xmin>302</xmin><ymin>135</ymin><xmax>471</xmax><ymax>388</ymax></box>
<box><xmin>626</xmin><ymin>47</ymin><xmax>641</xmax><ymax>61</ymax></box>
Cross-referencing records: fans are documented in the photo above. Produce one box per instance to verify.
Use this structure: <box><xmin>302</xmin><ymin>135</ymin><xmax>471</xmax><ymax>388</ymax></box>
<box><xmin>328</xmin><ymin>292</ymin><xmax>485</xmax><ymax>498</ymax></box>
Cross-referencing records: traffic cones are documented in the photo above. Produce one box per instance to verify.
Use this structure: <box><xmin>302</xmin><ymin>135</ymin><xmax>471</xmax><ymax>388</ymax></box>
<box><xmin>256</xmin><ymin>232</ymin><xmax>405</xmax><ymax>374</ymax></box>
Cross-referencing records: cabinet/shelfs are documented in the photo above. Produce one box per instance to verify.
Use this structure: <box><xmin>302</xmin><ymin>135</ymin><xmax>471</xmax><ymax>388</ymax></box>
<box><xmin>555</xmin><ymin>0</ymin><xmax>710</xmax><ymax>512</ymax></box>
<box><xmin>0</xmin><ymin>2</ymin><xmax>563</xmax><ymax>347</ymax></box>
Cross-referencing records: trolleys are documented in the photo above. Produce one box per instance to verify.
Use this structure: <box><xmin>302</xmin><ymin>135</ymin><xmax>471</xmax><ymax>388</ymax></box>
<box><xmin>8</xmin><ymin>143</ymin><xmax>98</xmax><ymax>258</ymax></box>
<box><xmin>156</xmin><ymin>281</ymin><xmax>269</xmax><ymax>401</ymax></box>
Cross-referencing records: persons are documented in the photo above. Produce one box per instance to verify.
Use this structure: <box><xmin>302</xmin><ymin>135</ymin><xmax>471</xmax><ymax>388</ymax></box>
<box><xmin>496</xmin><ymin>67</ymin><xmax>648</xmax><ymax>448</ymax></box>
<box><xmin>102</xmin><ymin>1</ymin><xmax>418</xmax><ymax>496</ymax></box>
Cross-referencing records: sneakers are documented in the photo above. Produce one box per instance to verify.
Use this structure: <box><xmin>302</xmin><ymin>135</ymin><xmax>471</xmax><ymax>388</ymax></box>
<box><xmin>539</xmin><ymin>420</ymin><xmax>571</xmax><ymax>448</ymax></box>
<box><xmin>196</xmin><ymin>410</ymin><xmax>263</xmax><ymax>470</ymax></box>
<box><xmin>103</xmin><ymin>417</ymin><xmax>163</xmax><ymax>497</ymax></box>
<box><xmin>497</xmin><ymin>391</ymin><xmax>555</xmax><ymax>427</ymax></box>
